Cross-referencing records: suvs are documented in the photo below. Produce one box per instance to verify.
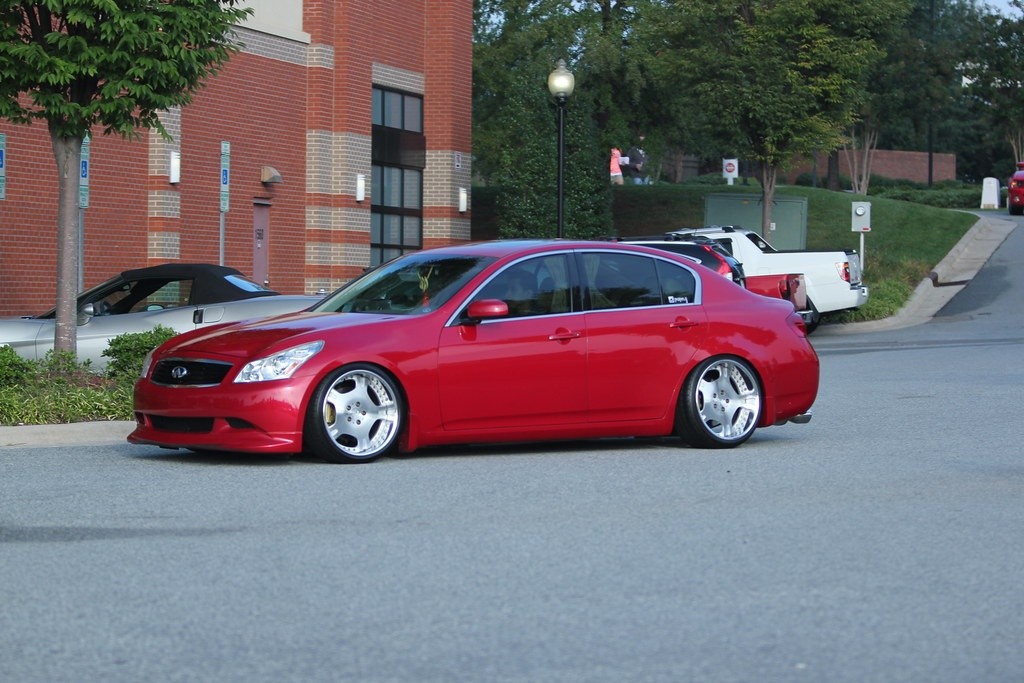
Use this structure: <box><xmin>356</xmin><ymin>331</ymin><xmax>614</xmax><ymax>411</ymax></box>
<box><xmin>615</xmin><ymin>240</ymin><xmax>745</xmax><ymax>292</ymax></box>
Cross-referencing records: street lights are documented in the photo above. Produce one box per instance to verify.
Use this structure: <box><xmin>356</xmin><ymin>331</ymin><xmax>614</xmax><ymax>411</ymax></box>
<box><xmin>548</xmin><ymin>58</ymin><xmax>576</xmax><ymax>239</ymax></box>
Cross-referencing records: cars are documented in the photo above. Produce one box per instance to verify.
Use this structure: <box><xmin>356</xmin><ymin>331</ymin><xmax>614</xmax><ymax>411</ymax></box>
<box><xmin>126</xmin><ymin>238</ymin><xmax>821</xmax><ymax>465</ymax></box>
<box><xmin>0</xmin><ymin>262</ymin><xmax>327</xmax><ymax>373</ymax></box>
<box><xmin>1008</xmin><ymin>162</ymin><xmax>1024</xmax><ymax>216</ymax></box>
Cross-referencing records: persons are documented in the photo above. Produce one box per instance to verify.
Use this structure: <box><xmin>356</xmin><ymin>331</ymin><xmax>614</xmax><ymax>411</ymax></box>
<box><xmin>507</xmin><ymin>270</ymin><xmax>537</xmax><ymax>313</ymax></box>
<box><xmin>627</xmin><ymin>136</ymin><xmax>651</xmax><ymax>184</ymax></box>
<box><xmin>611</xmin><ymin>147</ymin><xmax>625</xmax><ymax>184</ymax></box>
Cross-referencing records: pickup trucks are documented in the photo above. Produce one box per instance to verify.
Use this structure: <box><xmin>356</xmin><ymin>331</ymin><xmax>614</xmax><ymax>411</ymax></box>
<box><xmin>735</xmin><ymin>273</ymin><xmax>814</xmax><ymax>325</ymax></box>
<box><xmin>666</xmin><ymin>225</ymin><xmax>868</xmax><ymax>320</ymax></box>
